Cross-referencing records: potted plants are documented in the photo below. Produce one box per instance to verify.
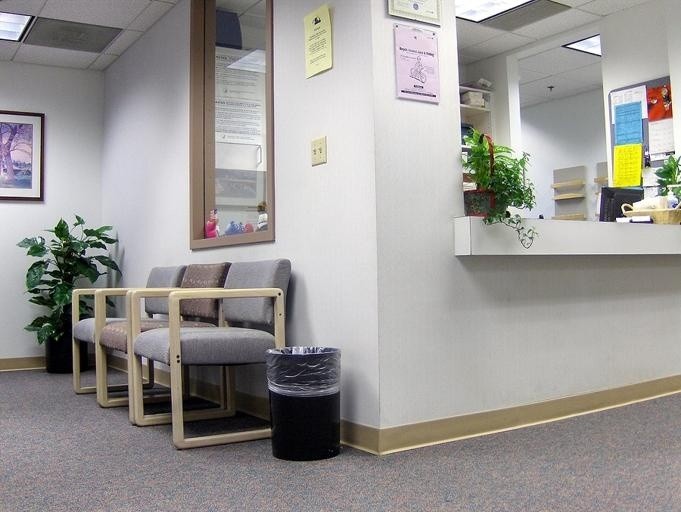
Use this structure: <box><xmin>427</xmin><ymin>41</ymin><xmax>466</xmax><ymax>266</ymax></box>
<box><xmin>17</xmin><ymin>212</ymin><xmax>124</xmax><ymax>373</ymax></box>
<box><xmin>461</xmin><ymin>125</ymin><xmax>540</xmax><ymax>248</ymax></box>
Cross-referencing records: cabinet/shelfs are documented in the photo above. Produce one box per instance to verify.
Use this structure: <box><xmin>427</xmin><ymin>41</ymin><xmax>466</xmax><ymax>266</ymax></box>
<box><xmin>550</xmin><ymin>179</ymin><xmax>589</xmax><ymax>220</ymax></box>
<box><xmin>459</xmin><ymin>83</ymin><xmax>491</xmax><ymax>114</ymax></box>
<box><xmin>593</xmin><ymin>176</ymin><xmax>607</xmax><ymax>221</ymax></box>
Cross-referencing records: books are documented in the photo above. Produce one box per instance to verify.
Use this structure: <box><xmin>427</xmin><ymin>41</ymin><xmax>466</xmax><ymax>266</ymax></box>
<box><xmin>463</xmin><ymin>164</ymin><xmax>478</xmax><ymax>192</ymax></box>
<box><xmin>460</xmin><ymin>90</ymin><xmax>484</xmax><ymax>107</ymax></box>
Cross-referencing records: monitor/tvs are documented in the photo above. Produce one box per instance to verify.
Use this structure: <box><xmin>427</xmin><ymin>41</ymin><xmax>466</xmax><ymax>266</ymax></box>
<box><xmin>600</xmin><ymin>186</ymin><xmax>644</xmax><ymax>222</ymax></box>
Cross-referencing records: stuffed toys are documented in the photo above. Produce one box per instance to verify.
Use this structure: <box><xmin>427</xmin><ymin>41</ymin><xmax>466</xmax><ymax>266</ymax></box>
<box><xmin>205</xmin><ymin>201</ymin><xmax>270</xmax><ymax>238</ymax></box>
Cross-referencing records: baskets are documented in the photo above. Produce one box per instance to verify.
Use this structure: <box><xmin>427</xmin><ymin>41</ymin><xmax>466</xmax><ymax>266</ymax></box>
<box><xmin>621</xmin><ymin>201</ymin><xmax>681</xmax><ymax>225</ymax></box>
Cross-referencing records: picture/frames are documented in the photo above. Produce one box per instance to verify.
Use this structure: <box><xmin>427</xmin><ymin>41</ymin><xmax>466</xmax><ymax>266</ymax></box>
<box><xmin>0</xmin><ymin>110</ymin><xmax>45</xmax><ymax>201</ymax></box>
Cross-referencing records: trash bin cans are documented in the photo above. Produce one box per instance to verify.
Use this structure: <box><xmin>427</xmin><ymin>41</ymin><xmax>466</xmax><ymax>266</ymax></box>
<box><xmin>266</xmin><ymin>346</ymin><xmax>341</xmax><ymax>461</ymax></box>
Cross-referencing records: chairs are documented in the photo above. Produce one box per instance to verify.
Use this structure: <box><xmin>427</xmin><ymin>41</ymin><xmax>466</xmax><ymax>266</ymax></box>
<box><xmin>68</xmin><ymin>263</ymin><xmax>190</xmax><ymax>394</ymax></box>
<box><xmin>95</xmin><ymin>263</ymin><xmax>233</xmax><ymax>408</ymax></box>
<box><xmin>131</xmin><ymin>257</ymin><xmax>292</xmax><ymax>449</ymax></box>
